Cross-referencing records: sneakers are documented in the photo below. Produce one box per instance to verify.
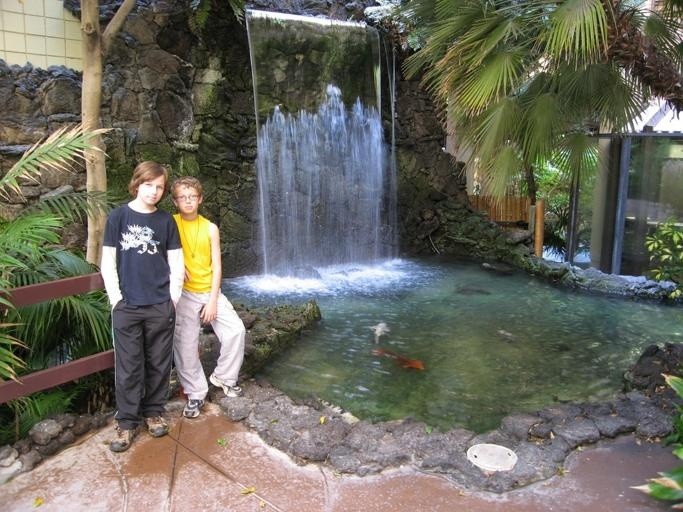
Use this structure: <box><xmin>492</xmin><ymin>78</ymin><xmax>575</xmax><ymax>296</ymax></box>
<box><xmin>110</xmin><ymin>423</ymin><xmax>140</xmax><ymax>451</ymax></box>
<box><xmin>144</xmin><ymin>414</ymin><xmax>170</xmax><ymax>438</ymax></box>
<box><xmin>208</xmin><ymin>371</ymin><xmax>244</xmax><ymax>398</ymax></box>
<box><xmin>182</xmin><ymin>399</ymin><xmax>205</xmax><ymax>419</ymax></box>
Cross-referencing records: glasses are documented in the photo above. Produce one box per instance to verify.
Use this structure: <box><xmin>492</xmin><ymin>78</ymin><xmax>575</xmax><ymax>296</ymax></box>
<box><xmin>174</xmin><ymin>194</ymin><xmax>200</xmax><ymax>203</ymax></box>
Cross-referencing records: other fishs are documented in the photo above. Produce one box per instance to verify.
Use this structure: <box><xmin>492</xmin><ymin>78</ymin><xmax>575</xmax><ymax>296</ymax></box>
<box><xmin>370</xmin><ymin>322</ymin><xmax>391</xmax><ymax>344</ymax></box>
<box><xmin>372</xmin><ymin>349</ymin><xmax>425</xmax><ymax>369</ymax></box>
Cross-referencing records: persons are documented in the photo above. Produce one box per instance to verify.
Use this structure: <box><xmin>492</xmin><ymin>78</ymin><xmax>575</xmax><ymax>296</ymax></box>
<box><xmin>101</xmin><ymin>160</ymin><xmax>185</xmax><ymax>452</ymax></box>
<box><xmin>170</xmin><ymin>176</ymin><xmax>246</xmax><ymax>418</ymax></box>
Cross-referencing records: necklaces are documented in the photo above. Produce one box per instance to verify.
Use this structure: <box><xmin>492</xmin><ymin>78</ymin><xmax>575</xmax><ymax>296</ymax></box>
<box><xmin>181</xmin><ymin>216</ymin><xmax>199</xmax><ymax>258</ymax></box>
